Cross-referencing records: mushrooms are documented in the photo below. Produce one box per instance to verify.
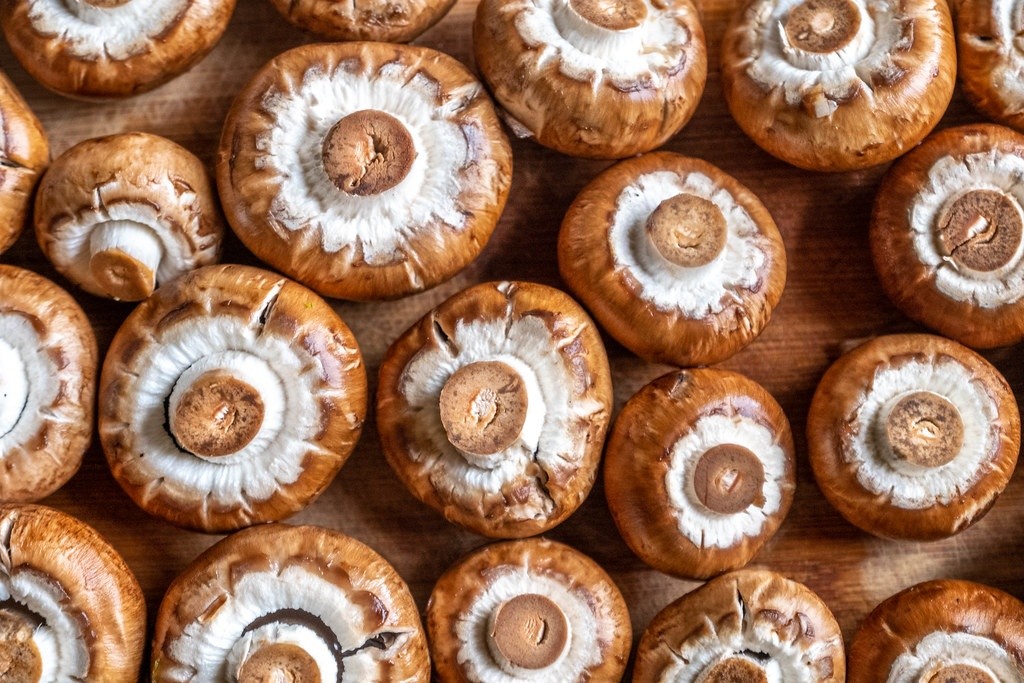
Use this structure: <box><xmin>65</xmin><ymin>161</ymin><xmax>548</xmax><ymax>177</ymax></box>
<box><xmin>0</xmin><ymin>0</ymin><xmax>1024</xmax><ymax>683</ymax></box>
